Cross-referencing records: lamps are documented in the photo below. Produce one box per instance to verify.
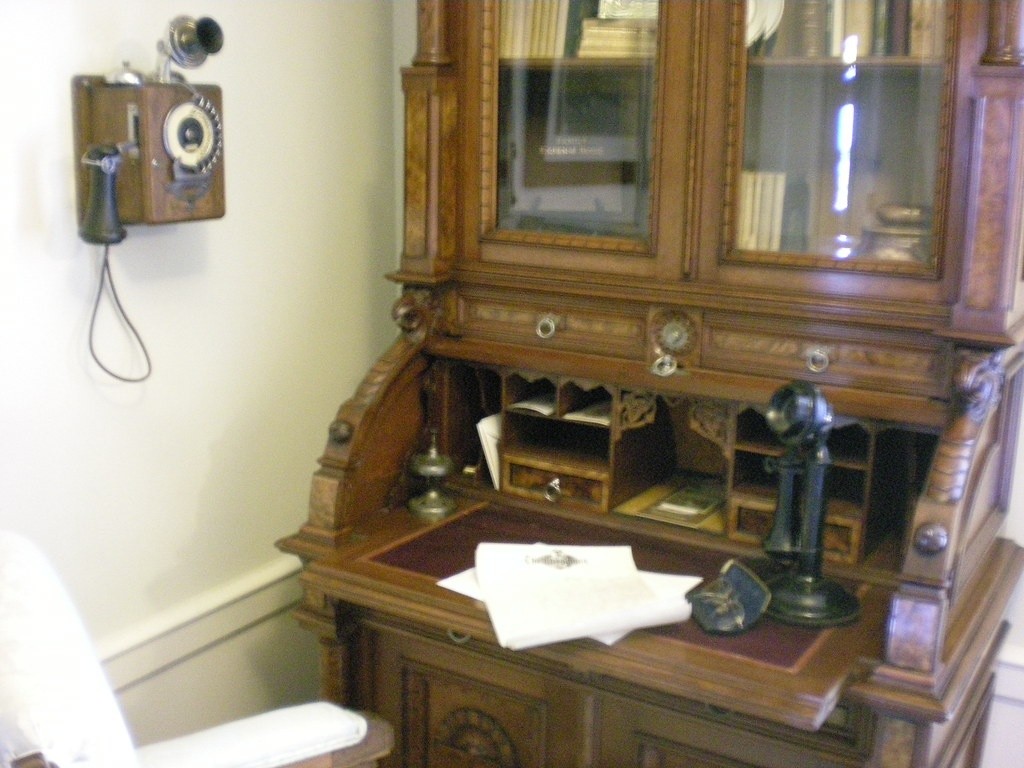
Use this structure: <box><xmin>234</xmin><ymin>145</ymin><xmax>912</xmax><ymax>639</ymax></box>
<box><xmin>765</xmin><ymin>381</ymin><xmax>861</xmax><ymax>625</ymax></box>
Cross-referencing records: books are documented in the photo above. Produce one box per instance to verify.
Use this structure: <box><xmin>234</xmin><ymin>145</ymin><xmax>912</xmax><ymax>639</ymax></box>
<box><xmin>746</xmin><ymin>0</ymin><xmax>946</xmax><ymax>61</ymax></box>
<box><xmin>499</xmin><ymin>0</ymin><xmax>659</xmax><ymax>59</ymax></box>
<box><xmin>734</xmin><ymin>170</ymin><xmax>787</xmax><ymax>250</ymax></box>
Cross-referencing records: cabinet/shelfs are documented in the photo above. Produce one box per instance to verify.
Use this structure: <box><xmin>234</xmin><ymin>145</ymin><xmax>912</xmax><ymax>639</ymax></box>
<box><xmin>274</xmin><ymin>0</ymin><xmax>1024</xmax><ymax>768</ymax></box>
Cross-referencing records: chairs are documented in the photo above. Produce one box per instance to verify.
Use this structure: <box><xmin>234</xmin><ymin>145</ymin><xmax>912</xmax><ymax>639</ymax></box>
<box><xmin>0</xmin><ymin>530</ymin><xmax>396</xmax><ymax>768</ymax></box>
